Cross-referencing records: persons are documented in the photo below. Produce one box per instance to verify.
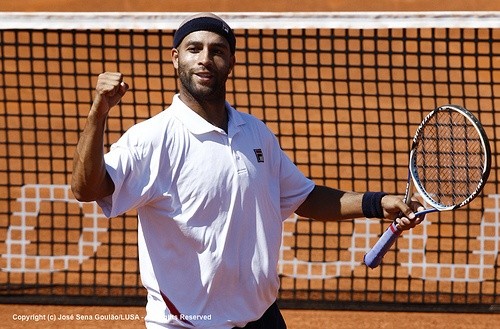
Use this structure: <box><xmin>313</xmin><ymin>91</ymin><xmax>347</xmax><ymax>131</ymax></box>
<box><xmin>71</xmin><ymin>13</ymin><xmax>426</xmax><ymax>329</ymax></box>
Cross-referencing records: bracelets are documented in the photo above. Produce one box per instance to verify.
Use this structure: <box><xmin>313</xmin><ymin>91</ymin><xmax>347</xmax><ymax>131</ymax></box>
<box><xmin>362</xmin><ymin>192</ymin><xmax>387</xmax><ymax>218</ymax></box>
